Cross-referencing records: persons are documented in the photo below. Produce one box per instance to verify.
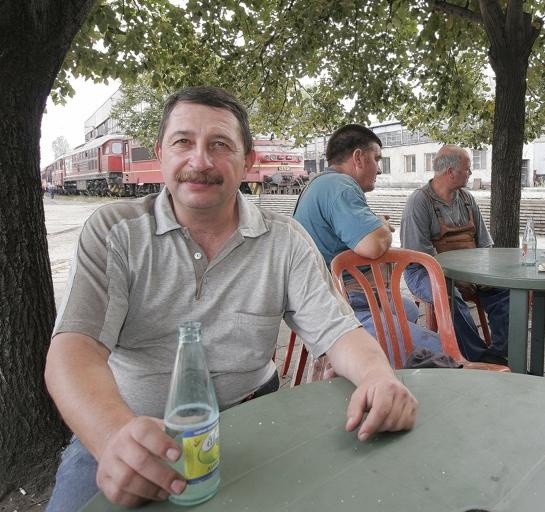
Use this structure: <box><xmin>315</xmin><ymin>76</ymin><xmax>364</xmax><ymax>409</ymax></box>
<box><xmin>293</xmin><ymin>122</ymin><xmax>450</xmax><ymax>369</ymax></box>
<box><xmin>400</xmin><ymin>146</ymin><xmax>509</xmax><ymax>366</ymax></box>
<box><xmin>43</xmin><ymin>86</ymin><xmax>421</xmax><ymax>511</ymax></box>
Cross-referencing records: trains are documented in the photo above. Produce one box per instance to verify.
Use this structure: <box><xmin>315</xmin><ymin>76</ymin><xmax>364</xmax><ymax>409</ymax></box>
<box><xmin>45</xmin><ymin>129</ymin><xmax>309</xmax><ymax>197</ymax></box>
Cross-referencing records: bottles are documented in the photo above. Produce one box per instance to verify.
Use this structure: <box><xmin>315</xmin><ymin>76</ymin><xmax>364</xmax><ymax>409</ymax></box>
<box><xmin>164</xmin><ymin>320</ymin><xmax>222</xmax><ymax>506</ymax></box>
<box><xmin>521</xmin><ymin>216</ymin><xmax>536</xmax><ymax>265</ymax></box>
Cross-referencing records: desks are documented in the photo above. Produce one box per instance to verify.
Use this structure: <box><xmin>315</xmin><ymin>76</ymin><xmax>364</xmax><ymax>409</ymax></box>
<box><xmin>77</xmin><ymin>368</ymin><xmax>545</xmax><ymax>512</ymax></box>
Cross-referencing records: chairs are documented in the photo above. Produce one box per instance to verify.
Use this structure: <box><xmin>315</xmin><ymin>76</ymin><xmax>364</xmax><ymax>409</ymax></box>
<box><xmin>279</xmin><ymin>248</ymin><xmax>545</xmax><ymax>388</ymax></box>
